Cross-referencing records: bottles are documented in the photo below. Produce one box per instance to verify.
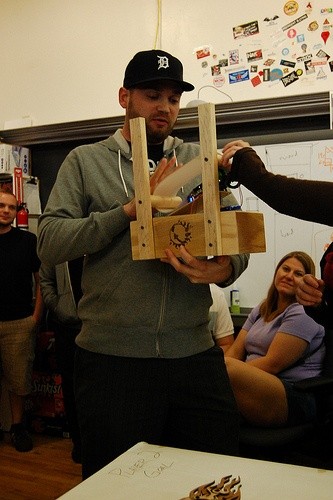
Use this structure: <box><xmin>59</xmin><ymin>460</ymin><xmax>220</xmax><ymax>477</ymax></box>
<box><xmin>230</xmin><ymin>290</ymin><xmax>241</xmax><ymax>315</ymax></box>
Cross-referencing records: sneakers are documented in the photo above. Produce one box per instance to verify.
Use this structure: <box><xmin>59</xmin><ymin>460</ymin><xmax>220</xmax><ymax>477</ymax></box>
<box><xmin>11</xmin><ymin>424</ymin><xmax>33</xmax><ymax>452</ymax></box>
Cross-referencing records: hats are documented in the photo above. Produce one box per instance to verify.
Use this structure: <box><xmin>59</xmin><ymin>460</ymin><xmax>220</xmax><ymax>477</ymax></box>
<box><xmin>123</xmin><ymin>50</ymin><xmax>194</xmax><ymax>91</ymax></box>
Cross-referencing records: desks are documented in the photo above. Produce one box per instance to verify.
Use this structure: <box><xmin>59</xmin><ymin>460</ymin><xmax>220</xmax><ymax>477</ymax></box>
<box><xmin>58</xmin><ymin>442</ymin><xmax>333</xmax><ymax>500</ymax></box>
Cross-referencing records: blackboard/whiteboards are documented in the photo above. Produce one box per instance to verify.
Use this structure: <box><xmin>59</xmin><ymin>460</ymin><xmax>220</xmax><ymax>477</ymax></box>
<box><xmin>188</xmin><ymin>130</ymin><xmax>333</xmax><ymax>326</ymax></box>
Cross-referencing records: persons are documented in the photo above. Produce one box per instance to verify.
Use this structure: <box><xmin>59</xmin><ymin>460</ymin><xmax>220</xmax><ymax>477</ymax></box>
<box><xmin>34</xmin><ymin>256</ymin><xmax>85</xmax><ymax>440</ymax></box>
<box><xmin>0</xmin><ymin>192</ymin><xmax>44</xmax><ymax>453</ymax></box>
<box><xmin>219</xmin><ymin>138</ymin><xmax>333</xmax><ymax>410</ymax></box>
<box><xmin>207</xmin><ymin>255</ymin><xmax>235</xmax><ymax>353</ymax></box>
<box><xmin>37</xmin><ymin>49</ymin><xmax>250</xmax><ymax>482</ymax></box>
<box><xmin>198</xmin><ymin>251</ymin><xmax>333</xmax><ymax>472</ymax></box>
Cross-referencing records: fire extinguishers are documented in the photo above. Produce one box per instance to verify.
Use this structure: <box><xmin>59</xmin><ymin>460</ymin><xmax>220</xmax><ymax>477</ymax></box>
<box><xmin>16</xmin><ymin>202</ymin><xmax>29</xmax><ymax>232</ymax></box>
